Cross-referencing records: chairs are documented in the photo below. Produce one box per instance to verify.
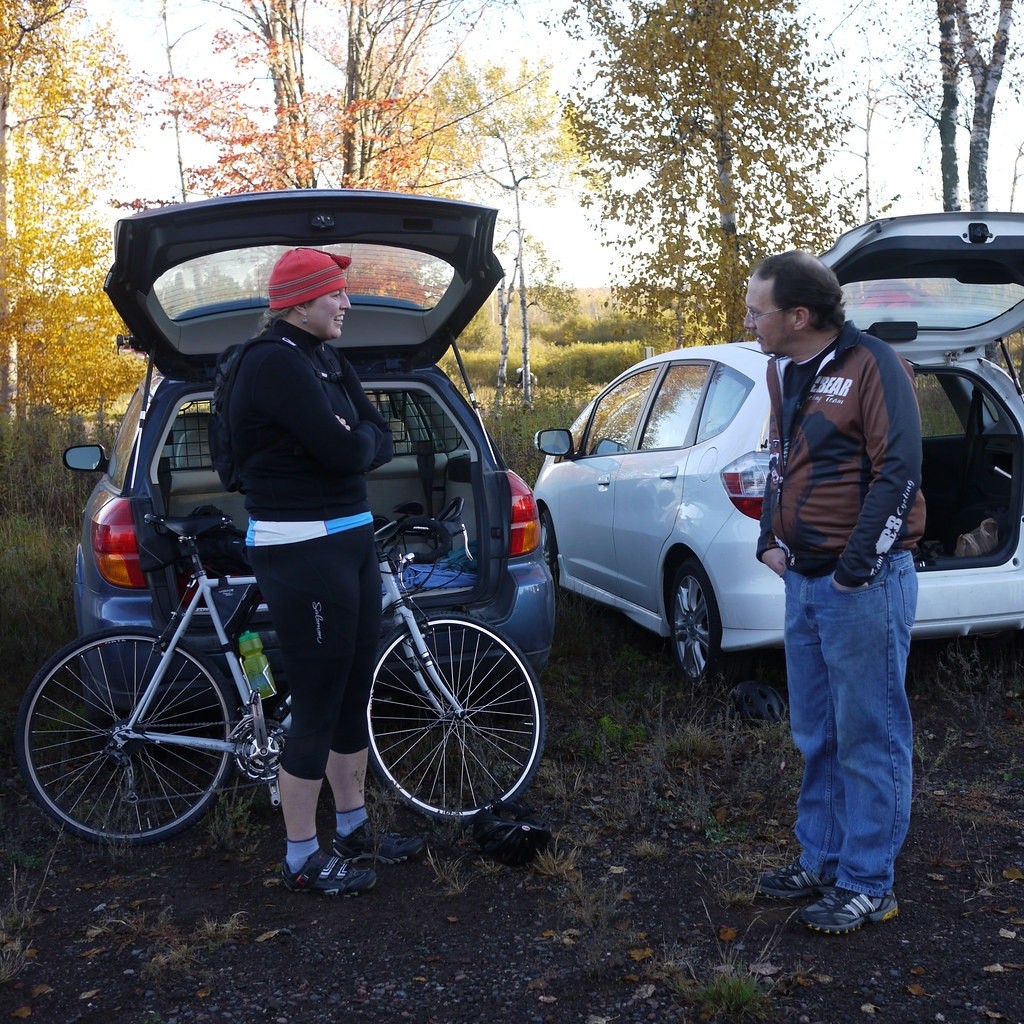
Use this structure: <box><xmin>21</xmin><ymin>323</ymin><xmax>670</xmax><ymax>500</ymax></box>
<box><xmin>166</xmin><ymin>412</ymin><xmax>211</xmax><ymax>470</ymax></box>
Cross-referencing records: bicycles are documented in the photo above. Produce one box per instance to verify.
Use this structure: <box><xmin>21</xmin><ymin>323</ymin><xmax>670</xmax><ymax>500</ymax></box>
<box><xmin>13</xmin><ymin>495</ymin><xmax>546</xmax><ymax>849</ymax></box>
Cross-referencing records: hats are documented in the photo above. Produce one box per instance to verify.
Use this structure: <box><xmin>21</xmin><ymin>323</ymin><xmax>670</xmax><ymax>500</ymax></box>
<box><xmin>269</xmin><ymin>248</ymin><xmax>352</xmax><ymax>309</ymax></box>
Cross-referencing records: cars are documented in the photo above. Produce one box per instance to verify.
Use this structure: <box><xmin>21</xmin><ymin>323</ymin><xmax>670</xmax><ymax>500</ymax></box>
<box><xmin>63</xmin><ymin>190</ymin><xmax>555</xmax><ymax>753</ymax></box>
<box><xmin>532</xmin><ymin>212</ymin><xmax>1024</xmax><ymax>688</ymax></box>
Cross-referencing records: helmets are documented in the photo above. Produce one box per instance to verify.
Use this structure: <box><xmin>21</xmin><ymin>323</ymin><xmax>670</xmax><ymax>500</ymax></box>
<box><xmin>474</xmin><ymin>802</ymin><xmax>549</xmax><ymax>867</ymax></box>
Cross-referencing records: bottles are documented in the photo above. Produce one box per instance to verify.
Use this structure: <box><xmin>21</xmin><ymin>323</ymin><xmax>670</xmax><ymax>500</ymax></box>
<box><xmin>239</xmin><ymin>631</ymin><xmax>277</xmax><ymax>699</ymax></box>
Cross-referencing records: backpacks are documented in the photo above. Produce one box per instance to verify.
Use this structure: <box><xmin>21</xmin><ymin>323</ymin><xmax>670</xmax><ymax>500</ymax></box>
<box><xmin>208</xmin><ymin>336</ymin><xmax>345</xmax><ymax>493</ymax></box>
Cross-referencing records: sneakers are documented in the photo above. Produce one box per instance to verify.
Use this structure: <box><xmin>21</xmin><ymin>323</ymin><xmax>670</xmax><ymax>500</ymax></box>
<box><xmin>332</xmin><ymin>817</ymin><xmax>425</xmax><ymax>866</ymax></box>
<box><xmin>794</xmin><ymin>886</ymin><xmax>899</xmax><ymax>935</ymax></box>
<box><xmin>281</xmin><ymin>849</ymin><xmax>378</xmax><ymax>898</ymax></box>
<box><xmin>754</xmin><ymin>859</ymin><xmax>838</xmax><ymax>897</ymax></box>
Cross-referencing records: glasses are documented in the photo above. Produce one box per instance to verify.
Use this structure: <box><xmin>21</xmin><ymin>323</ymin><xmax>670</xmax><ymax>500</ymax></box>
<box><xmin>745</xmin><ymin>302</ymin><xmax>814</xmax><ymax>322</ymax></box>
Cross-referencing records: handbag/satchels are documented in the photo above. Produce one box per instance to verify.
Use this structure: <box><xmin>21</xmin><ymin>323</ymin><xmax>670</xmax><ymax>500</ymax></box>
<box><xmin>954</xmin><ymin>517</ymin><xmax>999</xmax><ymax>557</ymax></box>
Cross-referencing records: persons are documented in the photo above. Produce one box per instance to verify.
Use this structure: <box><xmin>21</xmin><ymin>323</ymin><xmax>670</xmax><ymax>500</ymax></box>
<box><xmin>228</xmin><ymin>247</ymin><xmax>428</xmax><ymax>896</ymax></box>
<box><xmin>742</xmin><ymin>250</ymin><xmax>926</xmax><ymax>935</ymax></box>
